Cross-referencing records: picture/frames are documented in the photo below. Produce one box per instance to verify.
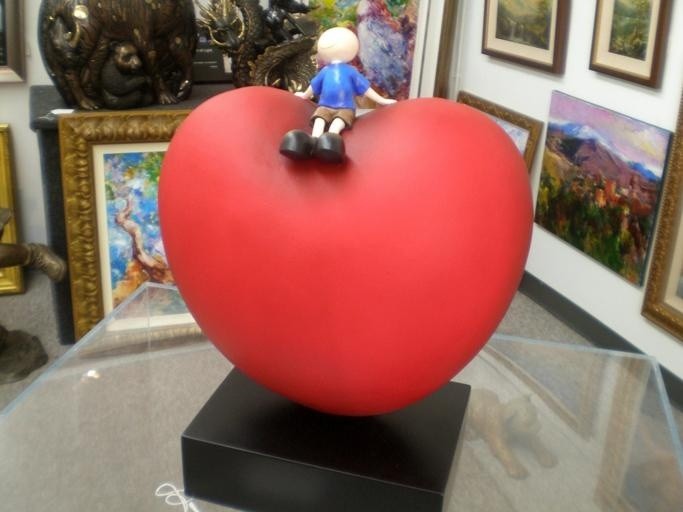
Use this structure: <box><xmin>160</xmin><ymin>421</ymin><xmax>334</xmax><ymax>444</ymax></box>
<box><xmin>271</xmin><ymin>0</ymin><xmax>452</xmax><ymax>120</ymax></box>
<box><xmin>593</xmin><ymin>356</ymin><xmax>682</xmax><ymax>512</ymax></box>
<box><xmin>457</xmin><ymin>89</ymin><xmax>545</xmax><ymax>172</ymax></box>
<box><xmin>641</xmin><ymin>89</ymin><xmax>683</xmax><ymax>342</ymax></box>
<box><xmin>589</xmin><ymin>0</ymin><xmax>671</xmax><ymax>88</ymax></box>
<box><xmin>484</xmin><ymin>333</ymin><xmax>609</xmax><ymax>443</ymax></box>
<box><xmin>1</xmin><ymin>123</ymin><xmax>26</xmax><ymax>296</ymax></box>
<box><xmin>58</xmin><ymin>110</ymin><xmax>205</xmax><ymax>360</ymax></box>
<box><xmin>481</xmin><ymin>0</ymin><xmax>571</xmax><ymax>74</ymax></box>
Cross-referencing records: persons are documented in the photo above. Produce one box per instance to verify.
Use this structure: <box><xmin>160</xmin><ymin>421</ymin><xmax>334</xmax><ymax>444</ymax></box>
<box><xmin>279</xmin><ymin>25</ymin><xmax>403</xmax><ymax>167</ymax></box>
